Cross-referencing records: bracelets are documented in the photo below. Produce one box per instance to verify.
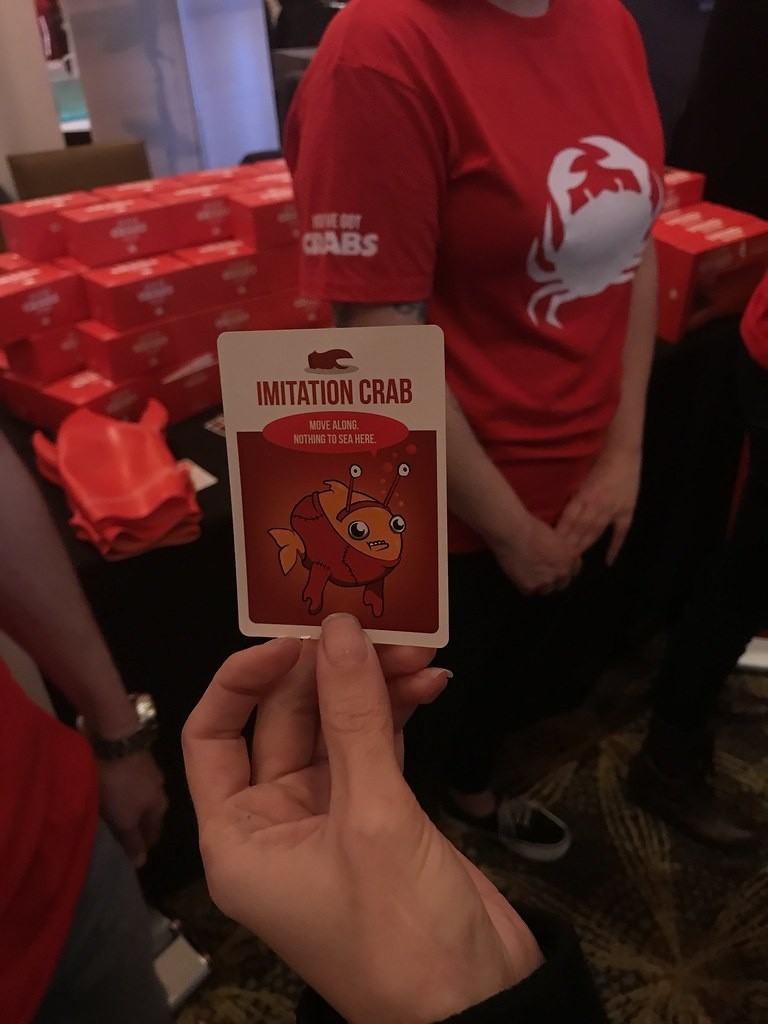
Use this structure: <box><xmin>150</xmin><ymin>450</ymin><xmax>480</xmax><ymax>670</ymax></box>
<box><xmin>75</xmin><ymin>694</ymin><xmax>158</xmax><ymax>759</ymax></box>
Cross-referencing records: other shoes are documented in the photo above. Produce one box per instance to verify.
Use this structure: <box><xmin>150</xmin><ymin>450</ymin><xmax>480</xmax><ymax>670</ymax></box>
<box><xmin>736</xmin><ymin>636</ymin><xmax>768</xmax><ymax>672</ymax></box>
<box><xmin>622</xmin><ymin>754</ymin><xmax>756</xmax><ymax>847</ymax></box>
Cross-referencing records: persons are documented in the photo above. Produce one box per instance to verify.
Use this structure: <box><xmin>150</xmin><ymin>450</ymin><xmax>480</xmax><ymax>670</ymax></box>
<box><xmin>279</xmin><ymin>0</ymin><xmax>667</xmax><ymax>859</ymax></box>
<box><xmin>0</xmin><ymin>431</ymin><xmax>170</xmax><ymax>1024</ymax></box>
<box><xmin>179</xmin><ymin>611</ymin><xmax>601</xmax><ymax>1024</ymax></box>
<box><xmin>620</xmin><ymin>268</ymin><xmax>768</xmax><ymax>856</ymax></box>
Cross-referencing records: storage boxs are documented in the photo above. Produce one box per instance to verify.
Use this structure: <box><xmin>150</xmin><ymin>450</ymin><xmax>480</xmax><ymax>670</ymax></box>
<box><xmin>0</xmin><ymin>156</ymin><xmax>768</xmax><ymax>432</ymax></box>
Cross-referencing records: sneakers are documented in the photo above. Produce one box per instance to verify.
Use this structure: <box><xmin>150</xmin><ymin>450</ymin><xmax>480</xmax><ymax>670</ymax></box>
<box><xmin>437</xmin><ymin>787</ymin><xmax>571</xmax><ymax>863</ymax></box>
